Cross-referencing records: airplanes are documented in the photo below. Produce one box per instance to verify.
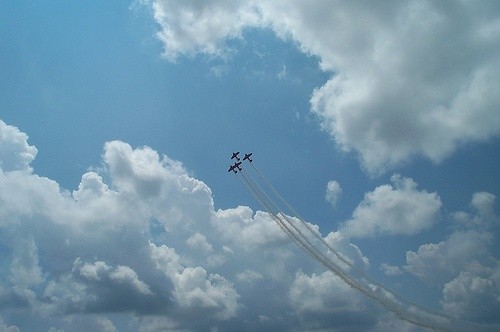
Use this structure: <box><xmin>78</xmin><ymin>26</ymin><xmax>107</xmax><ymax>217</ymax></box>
<box><xmin>228</xmin><ymin>165</ymin><xmax>237</xmax><ymax>173</ymax></box>
<box><xmin>243</xmin><ymin>152</ymin><xmax>253</xmax><ymax>163</ymax></box>
<box><xmin>235</xmin><ymin>162</ymin><xmax>242</xmax><ymax>170</ymax></box>
<box><xmin>231</xmin><ymin>152</ymin><xmax>240</xmax><ymax>161</ymax></box>
